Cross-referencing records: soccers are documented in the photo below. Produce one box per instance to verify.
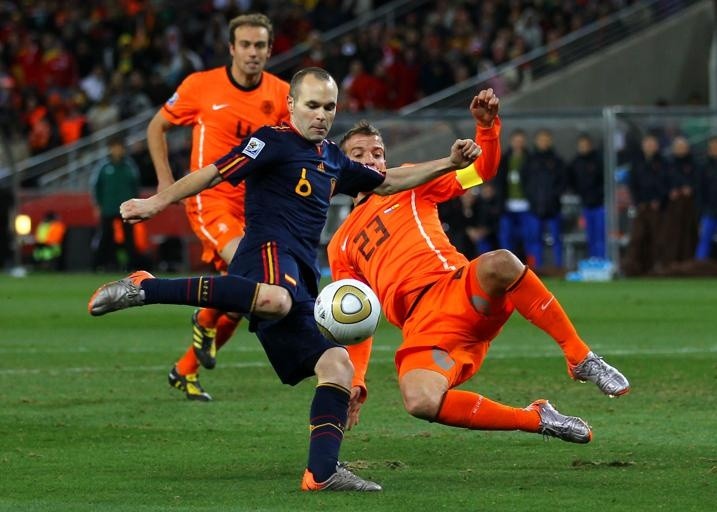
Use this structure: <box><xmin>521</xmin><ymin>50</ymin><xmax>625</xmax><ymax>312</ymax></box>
<box><xmin>315</xmin><ymin>278</ymin><xmax>380</xmax><ymax>345</ymax></box>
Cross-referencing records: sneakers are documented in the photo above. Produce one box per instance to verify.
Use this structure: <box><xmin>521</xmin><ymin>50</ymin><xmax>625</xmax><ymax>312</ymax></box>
<box><xmin>300</xmin><ymin>460</ymin><xmax>383</xmax><ymax>492</ymax></box>
<box><xmin>167</xmin><ymin>361</ymin><xmax>213</xmax><ymax>403</ymax></box>
<box><xmin>191</xmin><ymin>307</ymin><xmax>219</xmax><ymax>370</ymax></box>
<box><xmin>567</xmin><ymin>350</ymin><xmax>631</xmax><ymax>397</ymax></box>
<box><xmin>88</xmin><ymin>269</ymin><xmax>156</xmax><ymax>317</ymax></box>
<box><xmin>525</xmin><ymin>398</ymin><xmax>592</xmax><ymax>445</ymax></box>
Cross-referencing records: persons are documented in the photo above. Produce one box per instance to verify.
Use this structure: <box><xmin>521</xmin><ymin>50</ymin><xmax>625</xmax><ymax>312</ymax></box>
<box><xmin>327</xmin><ymin>88</ymin><xmax>631</xmax><ymax>443</ymax></box>
<box><xmin>89</xmin><ymin>65</ymin><xmax>483</xmax><ymax>492</ymax></box>
<box><xmin>148</xmin><ymin>14</ymin><xmax>290</xmax><ymax>401</ymax></box>
